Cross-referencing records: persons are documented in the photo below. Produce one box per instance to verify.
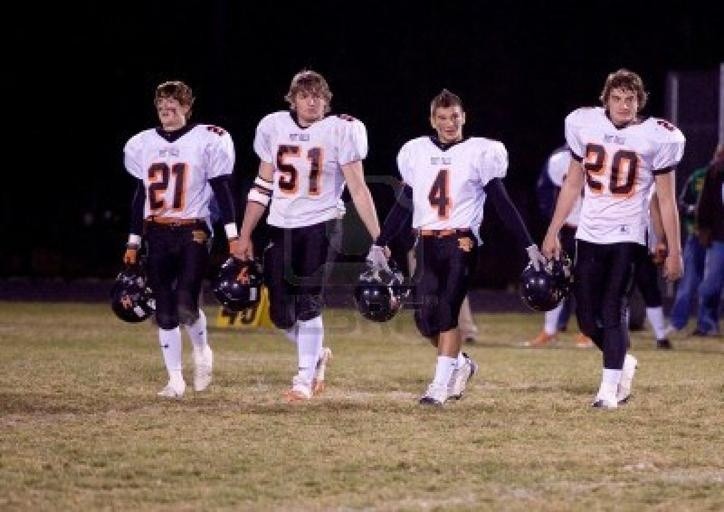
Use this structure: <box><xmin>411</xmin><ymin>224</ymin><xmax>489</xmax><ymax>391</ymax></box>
<box><xmin>235</xmin><ymin>71</ymin><xmax>391</xmax><ymax>404</ymax></box>
<box><xmin>408</xmin><ymin>250</ymin><xmax>477</xmax><ymax>346</ymax></box>
<box><xmin>541</xmin><ymin>71</ymin><xmax>687</xmax><ymax>409</ymax></box>
<box><xmin>366</xmin><ymin>89</ymin><xmax>547</xmax><ymax>408</ymax></box>
<box><xmin>524</xmin><ymin>135</ymin><xmax>723</xmax><ymax>351</ymax></box>
<box><xmin>124</xmin><ymin>80</ymin><xmax>240</xmax><ymax>399</ymax></box>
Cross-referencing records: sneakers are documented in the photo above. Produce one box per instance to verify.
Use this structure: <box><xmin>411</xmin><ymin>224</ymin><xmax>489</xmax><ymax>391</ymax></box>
<box><xmin>447</xmin><ymin>361</ymin><xmax>478</xmax><ymax>400</ymax></box>
<box><xmin>418</xmin><ymin>384</ymin><xmax>448</xmax><ymax>405</ymax></box>
<box><xmin>194</xmin><ymin>348</ymin><xmax>214</xmax><ymax>390</ymax></box>
<box><xmin>618</xmin><ymin>356</ymin><xmax>638</xmax><ymax>403</ymax></box>
<box><xmin>592</xmin><ymin>384</ymin><xmax>618</xmax><ymax>409</ymax></box>
<box><xmin>156</xmin><ymin>379</ymin><xmax>187</xmax><ymax>400</ymax></box>
<box><xmin>653</xmin><ymin>325</ymin><xmax>715</xmax><ymax>349</ymax></box>
<box><xmin>576</xmin><ymin>332</ymin><xmax>594</xmax><ymax>349</ymax></box>
<box><xmin>291</xmin><ymin>373</ymin><xmax>311</xmax><ymax>399</ymax></box>
<box><xmin>314</xmin><ymin>349</ymin><xmax>331</xmax><ymax>392</ymax></box>
<box><xmin>523</xmin><ymin>331</ymin><xmax>562</xmax><ymax>349</ymax></box>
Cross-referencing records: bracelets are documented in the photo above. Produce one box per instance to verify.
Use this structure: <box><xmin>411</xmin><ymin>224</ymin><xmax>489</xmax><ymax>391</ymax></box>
<box><xmin>224</xmin><ymin>221</ymin><xmax>239</xmax><ymax>240</ymax></box>
<box><xmin>127</xmin><ymin>234</ymin><xmax>142</xmax><ymax>247</ymax></box>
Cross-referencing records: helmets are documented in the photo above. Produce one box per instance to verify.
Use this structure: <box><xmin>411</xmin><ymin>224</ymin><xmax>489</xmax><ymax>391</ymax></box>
<box><xmin>213</xmin><ymin>257</ymin><xmax>265</xmax><ymax>312</ymax></box>
<box><xmin>353</xmin><ymin>266</ymin><xmax>410</xmax><ymax>320</ymax></box>
<box><xmin>110</xmin><ymin>266</ymin><xmax>155</xmax><ymax>323</ymax></box>
<box><xmin>518</xmin><ymin>254</ymin><xmax>574</xmax><ymax>314</ymax></box>
